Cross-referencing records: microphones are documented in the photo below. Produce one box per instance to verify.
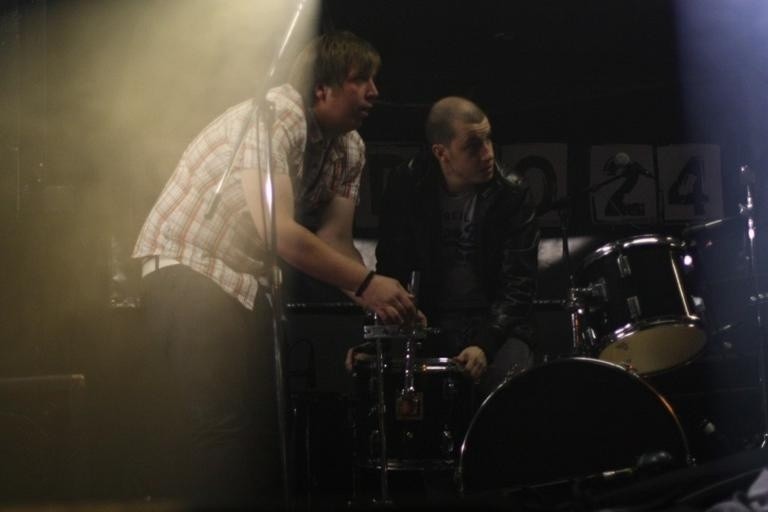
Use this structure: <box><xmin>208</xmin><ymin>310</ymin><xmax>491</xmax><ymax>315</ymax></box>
<box><xmin>739</xmin><ymin>165</ymin><xmax>757</xmax><ymax>240</ymax></box>
<box><xmin>615</xmin><ymin>153</ymin><xmax>652</xmax><ymax>177</ymax></box>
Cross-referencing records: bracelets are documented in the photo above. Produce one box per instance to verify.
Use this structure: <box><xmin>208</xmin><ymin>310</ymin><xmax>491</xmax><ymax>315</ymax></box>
<box><xmin>356</xmin><ymin>270</ymin><xmax>374</xmax><ymax>298</ymax></box>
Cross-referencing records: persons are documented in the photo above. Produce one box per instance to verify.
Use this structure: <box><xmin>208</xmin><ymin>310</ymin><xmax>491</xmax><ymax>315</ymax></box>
<box><xmin>130</xmin><ymin>30</ymin><xmax>417</xmax><ymax>510</ymax></box>
<box><xmin>376</xmin><ymin>97</ymin><xmax>542</xmax><ymax>507</ymax></box>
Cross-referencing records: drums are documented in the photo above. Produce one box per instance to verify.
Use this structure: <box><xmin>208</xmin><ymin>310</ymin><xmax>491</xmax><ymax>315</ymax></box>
<box><xmin>576</xmin><ymin>233</ymin><xmax>707</xmax><ymax>377</ymax></box>
<box><xmin>681</xmin><ymin>210</ymin><xmax>764</xmax><ymax>338</ymax></box>
<box><xmin>360</xmin><ymin>323</ymin><xmax>476</xmax><ymax>473</ymax></box>
<box><xmin>454</xmin><ymin>355</ymin><xmax>695</xmax><ymax>512</ymax></box>
